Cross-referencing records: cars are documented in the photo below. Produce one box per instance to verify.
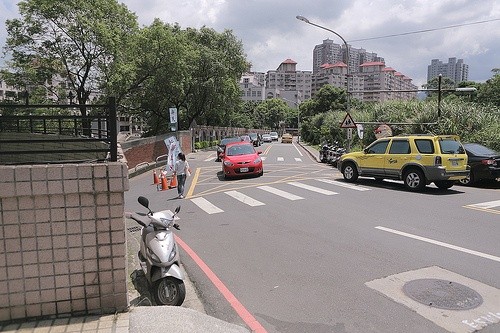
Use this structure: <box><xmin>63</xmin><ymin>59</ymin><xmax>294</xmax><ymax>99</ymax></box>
<box><xmin>240</xmin><ymin>136</ymin><xmax>253</xmax><ymax>144</ymax></box>
<box><xmin>458</xmin><ymin>143</ymin><xmax>500</xmax><ymax>186</ymax></box>
<box><xmin>259</xmin><ymin>135</ymin><xmax>272</xmax><ymax>143</ymax></box>
<box><xmin>219</xmin><ymin>142</ymin><xmax>263</xmax><ymax>180</ymax></box>
<box><xmin>217</xmin><ymin>138</ymin><xmax>244</xmax><ymax>160</ymax></box>
<box><xmin>282</xmin><ymin>134</ymin><xmax>292</xmax><ymax>143</ymax></box>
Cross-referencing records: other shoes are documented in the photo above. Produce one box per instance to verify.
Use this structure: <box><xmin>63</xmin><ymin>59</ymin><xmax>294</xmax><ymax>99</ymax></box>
<box><xmin>179</xmin><ymin>195</ymin><xmax>184</xmax><ymax>198</ymax></box>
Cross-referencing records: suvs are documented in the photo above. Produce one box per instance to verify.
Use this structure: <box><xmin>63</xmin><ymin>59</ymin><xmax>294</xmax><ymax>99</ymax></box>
<box><xmin>336</xmin><ymin>132</ymin><xmax>470</xmax><ymax>192</ymax></box>
<box><xmin>247</xmin><ymin>133</ymin><xmax>261</xmax><ymax>147</ymax></box>
<box><xmin>270</xmin><ymin>131</ymin><xmax>278</xmax><ymax>141</ymax></box>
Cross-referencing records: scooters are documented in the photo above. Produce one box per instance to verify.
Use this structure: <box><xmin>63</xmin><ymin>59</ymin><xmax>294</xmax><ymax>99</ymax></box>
<box><xmin>137</xmin><ymin>196</ymin><xmax>186</xmax><ymax>307</ymax></box>
<box><xmin>318</xmin><ymin>141</ymin><xmax>347</xmax><ymax>168</ymax></box>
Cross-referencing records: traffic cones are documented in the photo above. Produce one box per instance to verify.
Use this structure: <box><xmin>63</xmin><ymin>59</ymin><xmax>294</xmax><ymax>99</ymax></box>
<box><xmin>161</xmin><ymin>172</ymin><xmax>169</xmax><ymax>191</ymax></box>
<box><xmin>153</xmin><ymin>169</ymin><xmax>160</xmax><ymax>184</ymax></box>
<box><xmin>170</xmin><ymin>173</ymin><xmax>177</xmax><ymax>187</ymax></box>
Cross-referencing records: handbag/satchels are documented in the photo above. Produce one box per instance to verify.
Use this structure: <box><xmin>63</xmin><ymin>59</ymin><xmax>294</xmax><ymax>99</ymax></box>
<box><xmin>185</xmin><ymin>169</ymin><xmax>190</xmax><ymax>177</ymax></box>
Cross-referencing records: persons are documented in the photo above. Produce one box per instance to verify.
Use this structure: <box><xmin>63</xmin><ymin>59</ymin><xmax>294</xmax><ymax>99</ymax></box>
<box><xmin>174</xmin><ymin>153</ymin><xmax>188</xmax><ymax>198</ymax></box>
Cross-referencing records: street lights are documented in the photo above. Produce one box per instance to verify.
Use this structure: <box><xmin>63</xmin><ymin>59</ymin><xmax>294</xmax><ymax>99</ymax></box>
<box><xmin>282</xmin><ymin>97</ymin><xmax>300</xmax><ymax>143</ymax></box>
<box><xmin>295</xmin><ymin>15</ymin><xmax>352</xmax><ymax>153</ymax></box>
